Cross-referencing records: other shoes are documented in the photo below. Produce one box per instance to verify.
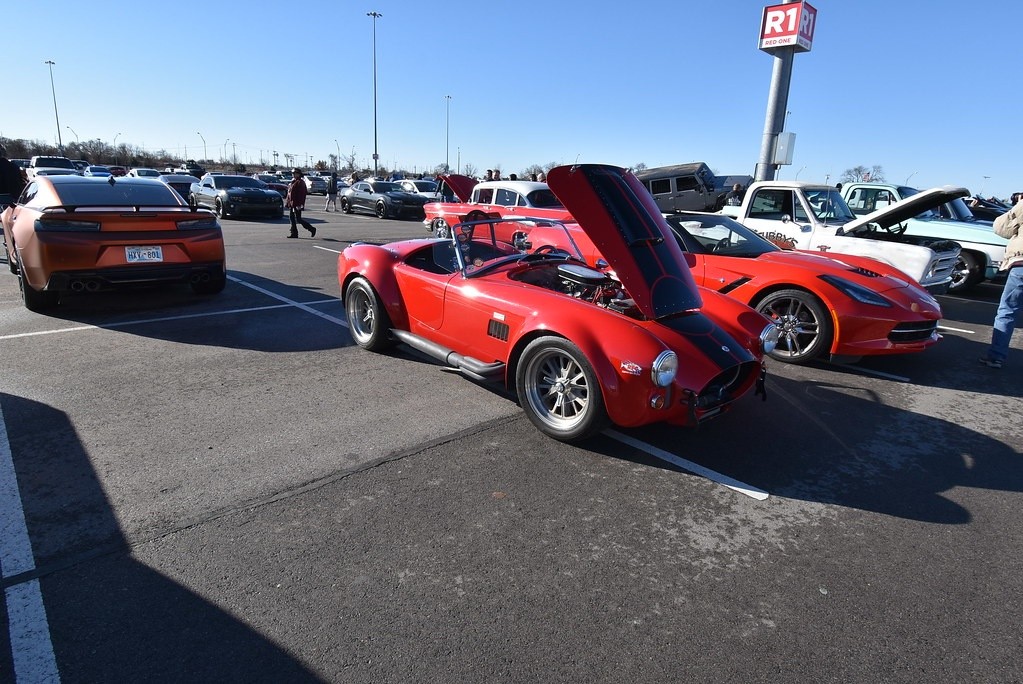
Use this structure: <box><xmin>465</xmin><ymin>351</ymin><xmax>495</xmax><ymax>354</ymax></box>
<box><xmin>334</xmin><ymin>209</ymin><xmax>338</xmax><ymax>211</ymax></box>
<box><xmin>310</xmin><ymin>227</ymin><xmax>317</xmax><ymax>237</ymax></box>
<box><xmin>286</xmin><ymin>234</ymin><xmax>298</xmax><ymax>238</ymax></box>
<box><xmin>325</xmin><ymin>209</ymin><xmax>329</xmax><ymax>212</ymax></box>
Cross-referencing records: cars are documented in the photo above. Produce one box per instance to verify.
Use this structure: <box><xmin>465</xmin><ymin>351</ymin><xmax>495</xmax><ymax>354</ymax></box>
<box><xmin>70</xmin><ymin>160</ymin><xmax>89</xmax><ymax>175</ymax></box>
<box><xmin>422</xmin><ymin>175</ymin><xmax>576</xmax><ymax>240</ymax></box>
<box><xmin>106</xmin><ymin>165</ymin><xmax>224</xmax><ymax>177</ymax></box>
<box><xmin>8</xmin><ymin>159</ymin><xmax>30</xmax><ymax>173</ymax></box>
<box><xmin>84</xmin><ymin>166</ymin><xmax>112</xmax><ymax>177</ymax></box>
<box><xmin>962</xmin><ymin>196</ymin><xmax>1012</xmax><ymax>223</ymax></box>
<box><xmin>392</xmin><ymin>181</ymin><xmax>460</xmax><ymax>203</ymax></box>
<box><xmin>251</xmin><ymin>170</ymin><xmax>384</xmax><ymax>196</ymax></box>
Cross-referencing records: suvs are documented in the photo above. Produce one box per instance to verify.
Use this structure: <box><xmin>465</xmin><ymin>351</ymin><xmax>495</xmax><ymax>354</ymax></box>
<box><xmin>632</xmin><ymin>163</ymin><xmax>753</xmax><ymax>213</ymax></box>
<box><xmin>25</xmin><ymin>155</ymin><xmax>78</xmax><ymax>184</ymax></box>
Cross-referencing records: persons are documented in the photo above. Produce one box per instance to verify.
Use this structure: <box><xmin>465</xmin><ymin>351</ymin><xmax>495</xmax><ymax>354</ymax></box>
<box><xmin>978</xmin><ymin>199</ymin><xmax>1023</xmax><ymax>367</ymax></box>
<box><xmin>485</xmin><ymin>169</ymin><xmax>546</xmax><ymax>183</ymax></box>
<box><xmin>726</xmin><ymin>184</ymin><xmax>744</xmax><ymax>206</ymax></box>
<box><xmin>285</xmin><ymin>168</ymin><xmax>316</xmax><ymax>238</ymax></box>
<box><xmin>325</xmin><ymin>172</ymin><xmax>338</xmax><ymax>211</ymax></box>
<box><xmin>967</xmin><ymin>200</ymin><xmax>978</xmax><ymax>213</ymax></box>
<box><xmin>404</xmin><ymin>174</ymin><xmax>422</xmax><ymax>180</ymax></box>
<box><xmin>434</xmin><ymin>175</ymin><xmax>454</xmax><ymax>203</ymax></box>
<box><xmin>0</xmin><ymin>144</ymin><xmax>17</xmax><ymax>208</ymax></box>
<box><xmin>386</xmin><ymin>173</ymin><xmax>397</xmax><ymax>182</ymax></box>
<box><xmin>352</xmin><ymin>172</ymin><xmax>358</xmax><ymax>184</ymax></box>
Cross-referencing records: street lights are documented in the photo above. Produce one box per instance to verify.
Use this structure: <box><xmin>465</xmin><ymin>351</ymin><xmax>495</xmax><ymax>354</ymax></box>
<box><xmin>45</xmin><ymin>60</ymin><xmax>62</xmax><ymax>144</ymax></box>
<box><xmin>445</xmin><ymin>96</ymin><xmax>451</xmax><ymax>173</ymax></box>
<box><xmin>367</xmin><ymin>11</ymin><xmax>382</xmax><ymax>176</ymax></box>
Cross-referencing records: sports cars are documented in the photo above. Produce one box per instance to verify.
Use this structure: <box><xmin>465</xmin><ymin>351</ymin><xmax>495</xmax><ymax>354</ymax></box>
<box><xmin>337</xmin><ymin>180</ymin><xmax>430</xmax><ymax>219</ymax></box>
<box><xmin>0</xmin><ymin>176</ymin><xmax>227</xmax><ymax>309</ymax></box>
<box><xmin>334</xmin><ymin>162</ymin><xmax>769</xmax><ymax>442</ymax></box>
<box><xmin>188</xmin><ymin>175</ymin><xmax>284</xmax><ymax>219</ymax></box>
<box><xmin>527</xmin><ymin>211</ymin><xmax>945</xmax><ymax>366</ymax></box>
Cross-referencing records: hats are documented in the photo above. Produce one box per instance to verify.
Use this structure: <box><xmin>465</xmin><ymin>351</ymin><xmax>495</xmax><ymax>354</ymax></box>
<box><xmin>291</xmin><ymin>168</ymin><xmax>304</xmax><ymax>178</ymax></box>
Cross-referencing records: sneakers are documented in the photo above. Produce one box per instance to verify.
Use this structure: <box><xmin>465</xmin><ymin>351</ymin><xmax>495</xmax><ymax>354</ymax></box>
<box><xmin>979</xmin><ymin>356</ymin><xmax>1002</xmax><ymax>368</ymax></box>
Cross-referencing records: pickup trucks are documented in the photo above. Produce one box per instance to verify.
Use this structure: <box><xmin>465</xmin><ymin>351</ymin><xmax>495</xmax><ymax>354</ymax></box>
<box><xmin>660</xmin><ymin>181</ymin><xmax>970</xmax><ymax>302</ymax></box>
<box><xmin>724</xmin><ymin>180</ymin><xmax>1016</xmax><ymax>290</ymax></box>
